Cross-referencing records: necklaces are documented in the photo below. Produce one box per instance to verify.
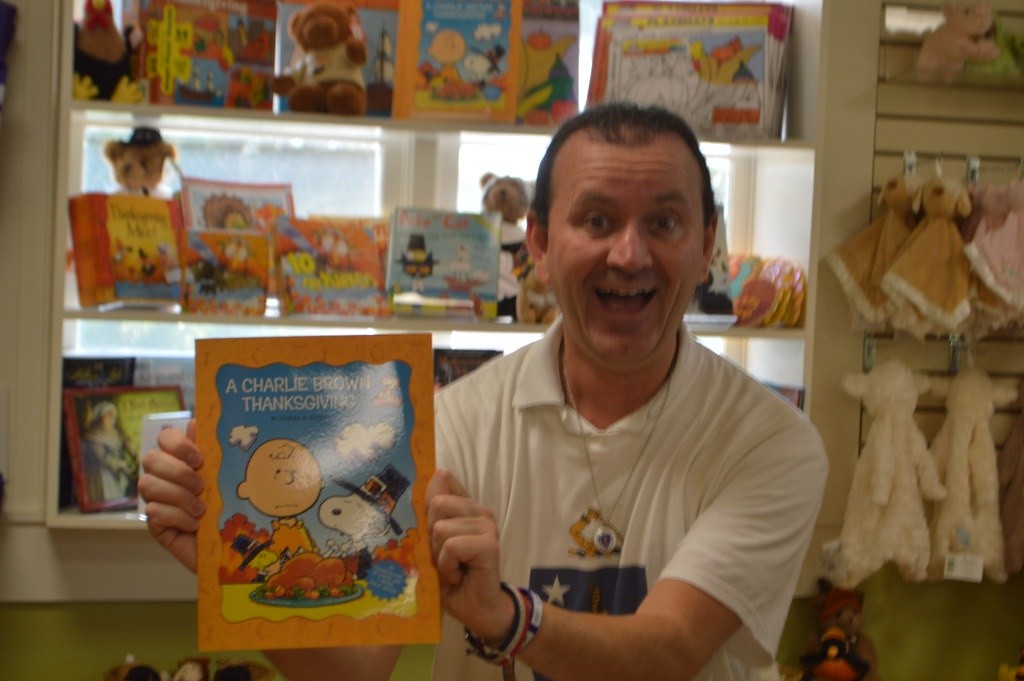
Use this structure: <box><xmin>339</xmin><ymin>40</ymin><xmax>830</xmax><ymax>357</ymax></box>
<box><xmin>560</xmin><ymin>354</ymin><xmax>669</xmax><ymax>554</ymax></box>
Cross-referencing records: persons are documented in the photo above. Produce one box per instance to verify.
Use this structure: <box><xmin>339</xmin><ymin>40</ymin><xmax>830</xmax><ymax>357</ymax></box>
<box><xmin>137</xmin><ymin>98</ymin><xmax>830</xmax><ymax>681</ymax></box>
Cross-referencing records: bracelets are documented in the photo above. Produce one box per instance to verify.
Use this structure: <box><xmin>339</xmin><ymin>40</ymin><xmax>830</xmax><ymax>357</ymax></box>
<box><xmin>463</xmin><ymin>581</ymin><xmax>541</xmax><ymax>666</ymax></box>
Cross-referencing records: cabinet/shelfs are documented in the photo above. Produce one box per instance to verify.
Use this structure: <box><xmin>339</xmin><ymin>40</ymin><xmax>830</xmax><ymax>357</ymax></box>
<box><xmin>44</xmin><ymin>0</ymin><xmax>831</xmax><ymax>530</ymax></box>
